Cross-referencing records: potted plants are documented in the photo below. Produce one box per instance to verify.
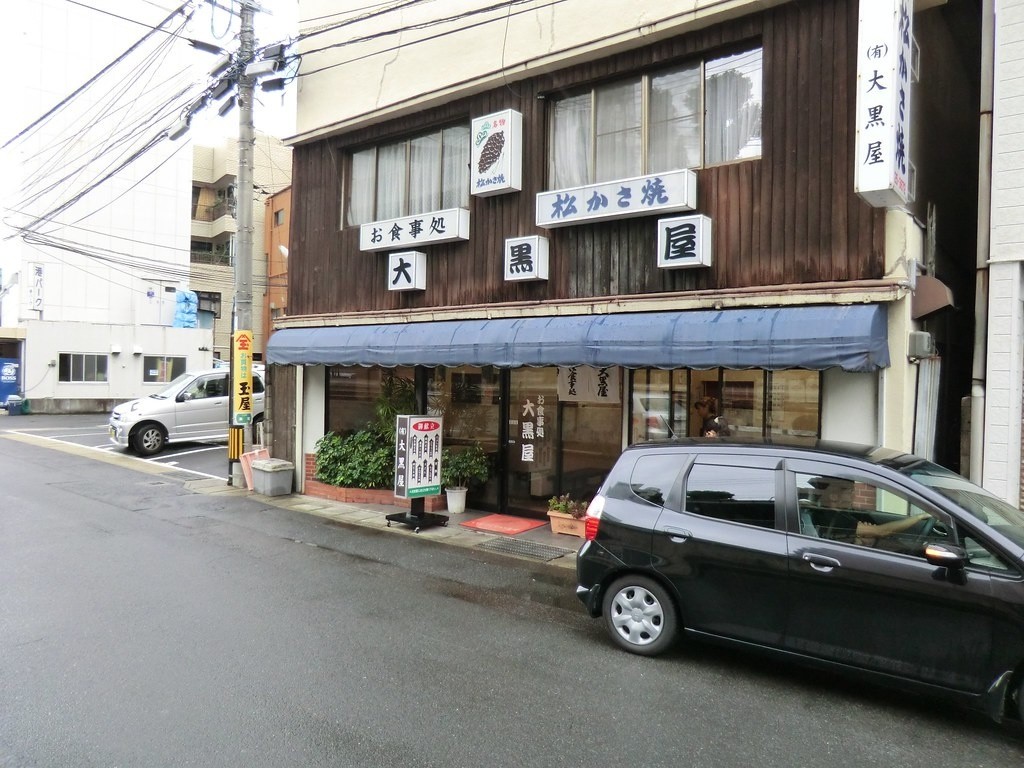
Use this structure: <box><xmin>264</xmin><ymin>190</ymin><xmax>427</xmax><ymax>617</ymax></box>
<box><xmin>440</xmin><ymin>439</ymin><xmax>489</xmax><ymax>513</ymax></box>
<box><xmin>546</xmin><ymin>494</ymin><xmax>587</xmax><ymax>540</ymax></box>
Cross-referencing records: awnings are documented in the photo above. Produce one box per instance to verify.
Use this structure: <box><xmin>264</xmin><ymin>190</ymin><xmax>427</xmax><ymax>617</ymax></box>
<box><xmin>265</xmin><ymin>306</ymin><xmax>889</xmax><ymax>371</ymax></box>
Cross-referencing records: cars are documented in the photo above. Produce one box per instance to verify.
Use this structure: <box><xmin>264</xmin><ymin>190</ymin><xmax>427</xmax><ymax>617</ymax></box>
<box><xmin>109</xmin><ymin>363</ymin><xmax>265</xmax><ymax>458</ymax></box>
<box><xmin>574</xmin><ymin>436</ymin><xmax>1024</xmax><ymax>727</ymax></box>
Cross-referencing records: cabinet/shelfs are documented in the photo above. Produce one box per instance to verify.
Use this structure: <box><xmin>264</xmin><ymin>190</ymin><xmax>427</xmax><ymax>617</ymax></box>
<box><xmin>638</xmin><ymin>393</ymin><xmax>689</xmax><ymax>439</ymax></box>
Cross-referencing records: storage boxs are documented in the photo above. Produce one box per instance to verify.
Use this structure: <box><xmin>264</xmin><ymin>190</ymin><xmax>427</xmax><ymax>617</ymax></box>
<box><xmin>250</xmin><ymin>459</ymin><xmax>296</xmax><ymax>496</ymax></box>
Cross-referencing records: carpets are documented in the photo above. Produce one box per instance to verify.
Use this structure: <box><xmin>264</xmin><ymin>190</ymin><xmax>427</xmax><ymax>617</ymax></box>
<box><xmin>459</xmin><ymin>512</ymin><xmax>550</xmax><ymax>535</ymax></box>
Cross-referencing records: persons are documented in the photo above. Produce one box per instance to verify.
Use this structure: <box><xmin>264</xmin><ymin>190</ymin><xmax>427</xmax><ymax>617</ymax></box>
<box><xmin>695</xmin><ymin>397</ymin><xmax>730</xmax><ymax>437</ymax></box>
<box><xmin>190</xmin><ymin>379</ymin><xmax>207</xmax><ymax>399</ymax></box>
<box><xmin>812</xmin><ymin>480</ymin><xmax>934</xmax><ymax>545</ymax></box>
